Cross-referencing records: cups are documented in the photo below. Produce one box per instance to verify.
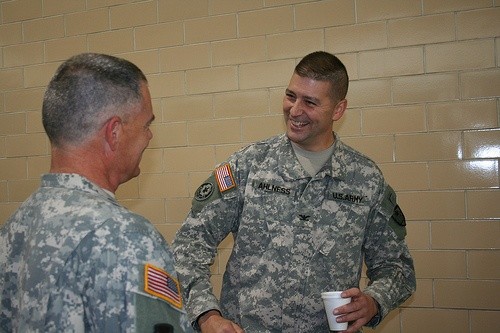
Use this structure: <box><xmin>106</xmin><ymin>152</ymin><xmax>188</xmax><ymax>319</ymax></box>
<box><xmin>321</xmin><ymin>292</ymin><xmax>351</xmax><ymax>330</ymax></box>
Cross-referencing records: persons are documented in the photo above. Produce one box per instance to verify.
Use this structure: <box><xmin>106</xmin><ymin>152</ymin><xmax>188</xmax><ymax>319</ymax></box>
<box><xmin>1</xmin><ymin>52</ymin><xmax>191</xmax><ymax>333</ymax></box>
<box><xmin>172</xmin><ymin>51</ymin><xmax>416</xmax><ymax>333</ymax></box>
